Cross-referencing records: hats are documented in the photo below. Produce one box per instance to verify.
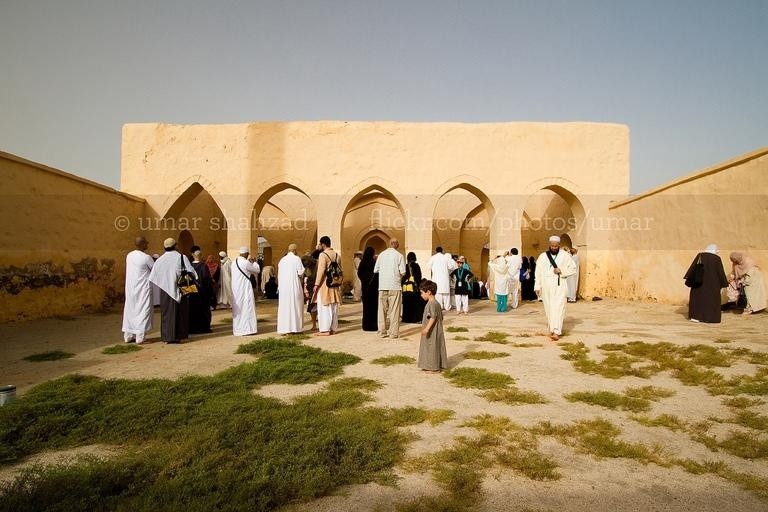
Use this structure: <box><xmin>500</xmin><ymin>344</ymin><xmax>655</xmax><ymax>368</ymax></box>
<box><xmin>549</xmin><ymin>236</ymin><xmax>560</xmax><ymax>243</ymax></box>
<box><xmin>240</xmin><ymin>247</ymin><xmax>249</xmax><ymax>254</ymax></box>
<box><xmin>163</xmin><ymin>238</ymin><xmax>176</xmax><ymax>249</ymax></box>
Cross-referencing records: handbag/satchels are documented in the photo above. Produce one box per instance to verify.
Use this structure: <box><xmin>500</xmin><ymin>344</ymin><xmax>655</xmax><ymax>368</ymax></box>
<box><xmin>250</xmin><ymin>274</ymin><xmax>256</xmax><ymax>288</ymax></box>
<box><xmin>685</xmin><ymin>254</ymin><xmax>703</xmax><ymax>288</ymax></box>
<box><xmin>178</xmin><ymin>271</ymin><xmax>200</xmax><ymax>295</ymax></box>
<box><xmin>736</xmin><ymin>284</ymin><xmax>747</xmax><ymax>309</ymax></box>
<box><xmin>727</xmin><ymin>280</ymin><xmax>738</xmax><ymax>302</ymax></box>
<box><xmin>403</xmin><ymin>276</ymin><xmax>417</xmax><ymax>295</ymax></box>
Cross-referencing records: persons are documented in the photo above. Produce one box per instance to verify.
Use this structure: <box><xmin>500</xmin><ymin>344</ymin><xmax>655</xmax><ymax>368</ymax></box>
<box><xmin>726</xmin><ymin>252</ymin><xmax>768</xmax><ymax>315</ymax></box>
<box><xmin>120</xmin><ymin>235</ymin><xmax>580</xmax><ymax>377</ymax></box>
<box><xmin>683</xmin><ymin>243</ymin><xmax>729</xmax><ymax>323</ymax></box>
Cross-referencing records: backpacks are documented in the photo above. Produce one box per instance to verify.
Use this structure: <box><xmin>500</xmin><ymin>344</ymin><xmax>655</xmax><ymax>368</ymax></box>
<box><xmin>322</xmin><ymin>251</ymin><xmax>343</xmax><ymax>287</ymax></box>
